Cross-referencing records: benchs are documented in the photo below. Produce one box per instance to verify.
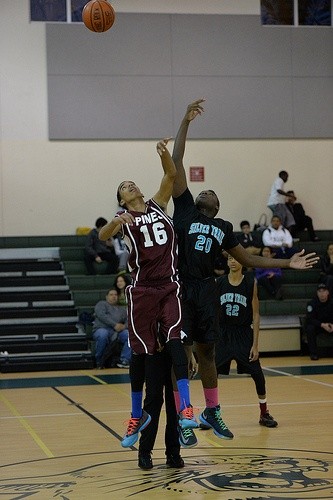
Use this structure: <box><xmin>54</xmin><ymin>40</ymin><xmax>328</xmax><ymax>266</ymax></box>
<box><xmin>58</xmin><ymin>238</ymin><xmax>333</xmax><ymax>354</ymax></box>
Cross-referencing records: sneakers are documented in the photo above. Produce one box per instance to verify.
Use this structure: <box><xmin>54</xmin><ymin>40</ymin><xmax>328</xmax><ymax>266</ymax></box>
<box><xmin>178</xmin><ymin>398</ymin><xmax>200</xmax><ymax>429</ymax></box>
<box><xmin>259</xmin><ymin>410</ymin><xmax>277</xmax><ymax>427</ymax></box>
<box><xmin>121</xmin><ymin>408</ymin><xmax>151</xmax><ymax>448</ymax></box>
<box><xmin>200</xmin><ymin>403</ymin><xmax>234</xmax><ymax>440</ymax></box>
<box><xmin>178</xmin><ymin>429</ymin><xmax>199</xmax><ymax>448</ymax></box>
<box><xmin>199</xmin><ymin>423</ymin><xmax>212</xmax><ymax>430</ymax></box>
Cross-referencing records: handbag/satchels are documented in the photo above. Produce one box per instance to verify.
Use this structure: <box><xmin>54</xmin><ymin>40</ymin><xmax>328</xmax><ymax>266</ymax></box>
<box><xmin>253</xmin><ymin>214</ymin><xmax>267</xmax><ymax>231</ymax></box>
<box><xmin>76</xmin><ymin>225</ymin><xmax>92</xmax><ymax>235</ymax></box>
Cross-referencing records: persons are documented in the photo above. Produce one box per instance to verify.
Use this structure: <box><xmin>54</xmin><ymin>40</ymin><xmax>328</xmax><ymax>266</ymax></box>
<box><xmin>84</xmin><ymin>215</ymin><xmax>117</xmax><ymax>274</ymax></box>
<box><xmin>90</xmin><ymin>287</ymin><xmax>131</xmax><ymax>372</ymax></box>
<box><xmin>112</xmin><ymin>273</ymin><xmax>132</xmax><ymax>311</ymax></box>
<box><xmin>253</xmin><ymin>246</ymin><xmax>283</xmax><ymax>299</ymax></box>
<box><xmin>286</xmin><ymin>190</ymin><xmax>320</xmax><ymax>244</ymax></box>
<box><xmin>268</xmin><ymin>169</ymin><xmax>298</xmax><ymax>232</ymax></box>
<box><xmin>96</xmin><ymin>136</ymin><xmax>202</xmax><ymax>448</ymax></box>
<box><xmin>235</xmin><ymin>220</ymin><xmax>262</xmax><ymax>256</ymax></box>
<box><xmin>166</xmin><ymin>93</ymin><xmax>319</xmax><ymax>448</ymax></box>
<box><xmin>319</xmin><ymin>243</ymin><xmax>333</xmax><ymax>288</ymax></box>
<box><xmin>137</xmin><ymin>353</ymin><xmax>187</xmax><ymax>472</ymax></box>
<box><xmin>201</xmin><ymin>251</ymin><xmax>281</xmax><ymax>432</ymax></box>
<box><xmin>262</xmin><ymin>215</ymin><xmax>299</xmax><ymax>261</ymax></box>
<box><xmin>112</xmin><ymin>233</ymin><xmax>131</xmax><ymax>274</ymax></box>
<box><xmin>304</xmin><ymin>283</ymin><xmax>333</xmax><ymax>361</ymax></box>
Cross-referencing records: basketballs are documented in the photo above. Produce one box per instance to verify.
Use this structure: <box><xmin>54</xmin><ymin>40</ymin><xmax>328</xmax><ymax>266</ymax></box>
<box><xmin>82</xmin><ymin>0</ymin><xmax>116</xmax><ymax>33</ymax></box>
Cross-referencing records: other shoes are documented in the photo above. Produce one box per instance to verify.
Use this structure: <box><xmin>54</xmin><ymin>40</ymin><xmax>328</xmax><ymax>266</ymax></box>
<box><xmin>117</xmin><ymin>269</ymin><xmax>127</xmax><ymax>275</ymax></box>
<box><xmin>311</xmin><ymin>354</ymin><xmax>319</xmax><ymax>361</ymax></box>
<box><xmin>117</xmin><ymin>358</ymin><xmax>130</xmax><ymax>369</ymax></box>
